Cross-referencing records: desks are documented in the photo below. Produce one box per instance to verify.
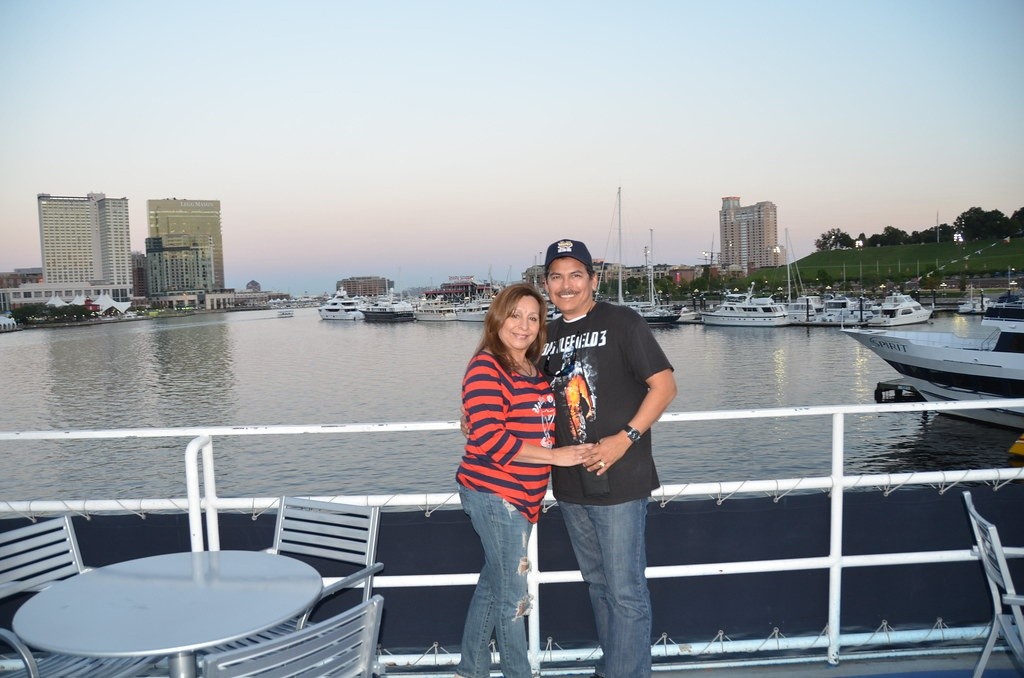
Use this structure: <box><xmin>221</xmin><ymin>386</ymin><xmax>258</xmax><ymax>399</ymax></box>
<box><xmin>14</xmin><ymin>549</ymin><xmax>322</xmax><ymax>678</ymax></box>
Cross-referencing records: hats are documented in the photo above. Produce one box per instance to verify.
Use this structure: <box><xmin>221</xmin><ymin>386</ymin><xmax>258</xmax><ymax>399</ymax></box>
<box><xmin>545</xmin><ymin>239</ymin><xmax>593</xmax><ymax>268</ymax></box>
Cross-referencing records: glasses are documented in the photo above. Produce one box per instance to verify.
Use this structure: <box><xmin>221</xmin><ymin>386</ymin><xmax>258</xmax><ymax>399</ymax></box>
<box><xmin>544</xmin><ymin>348</ymin><xmax>577</xmax><ymax>377</ymax></box>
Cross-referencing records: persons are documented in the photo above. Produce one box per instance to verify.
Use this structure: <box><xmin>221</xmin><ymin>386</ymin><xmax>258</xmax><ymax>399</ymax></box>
<box><xmin>454</xmin><ymin>283</ymin><xmax>595</xmax><ymax>678</ymax></box>
<box><xmin>460</xmin><ymin>239</ymin><xmax>679</xmax><ymax>678</ymax></box>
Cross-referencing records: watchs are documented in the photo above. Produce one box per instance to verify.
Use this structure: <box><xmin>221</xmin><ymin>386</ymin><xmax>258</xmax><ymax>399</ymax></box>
<box><xmin>623</xmin><ymin>425</ymin><xmax>641</xmax><ymax>442</ymax></box>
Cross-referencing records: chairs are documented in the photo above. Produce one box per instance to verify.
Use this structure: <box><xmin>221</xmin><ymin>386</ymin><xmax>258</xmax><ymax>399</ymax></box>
<box><xmin>962</xmin><ymin>490</ymin><xmax>1024</xmax><ymax>678</ymax></box>
<box><xmin>200</xmin><ymin>497</ymin><xmax>385</xmax><ymax>678</ymax></box>
<box><xmin>1</xmin><ymin>512</ymin><xmax>172</xmax><ymax>678</ymax></box>
<box><xmin>200</xmin><ymin>591</ymin><xmax>386</xmax><ymax>678</ymax></box>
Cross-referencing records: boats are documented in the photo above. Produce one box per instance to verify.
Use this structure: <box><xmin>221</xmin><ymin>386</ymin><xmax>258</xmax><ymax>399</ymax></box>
<box><xmin>316</xmin><ymin>186</ymin><xmax>1024</xmax><ymax>328</ymax></box>
<box><xmin>277</xmin><ymin>308</ymin><xmax>295</xmax><ymax>319</ymax></box>
<box><xmin>840</xmin><ymin>323</ymin><xmax>1024</xmax><ymax>433</ymax></box>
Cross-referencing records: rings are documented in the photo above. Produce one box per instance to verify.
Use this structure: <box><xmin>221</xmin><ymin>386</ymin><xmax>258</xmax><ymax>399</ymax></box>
<box><xmin>598</xmin><ymin>461</ymin><xmax>605</xmax><ymax>467</ymax></box>
<box><xmin>598</xmin><ymin>441</ymin><xmax>600</xmax><ymax>444</ymax></box>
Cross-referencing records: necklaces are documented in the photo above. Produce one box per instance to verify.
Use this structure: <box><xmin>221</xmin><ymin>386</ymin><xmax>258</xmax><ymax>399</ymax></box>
<box><xmin>511</xmin><ymin>356</ymin><xmax>533</xmax><ymax>377</ymax></box>
